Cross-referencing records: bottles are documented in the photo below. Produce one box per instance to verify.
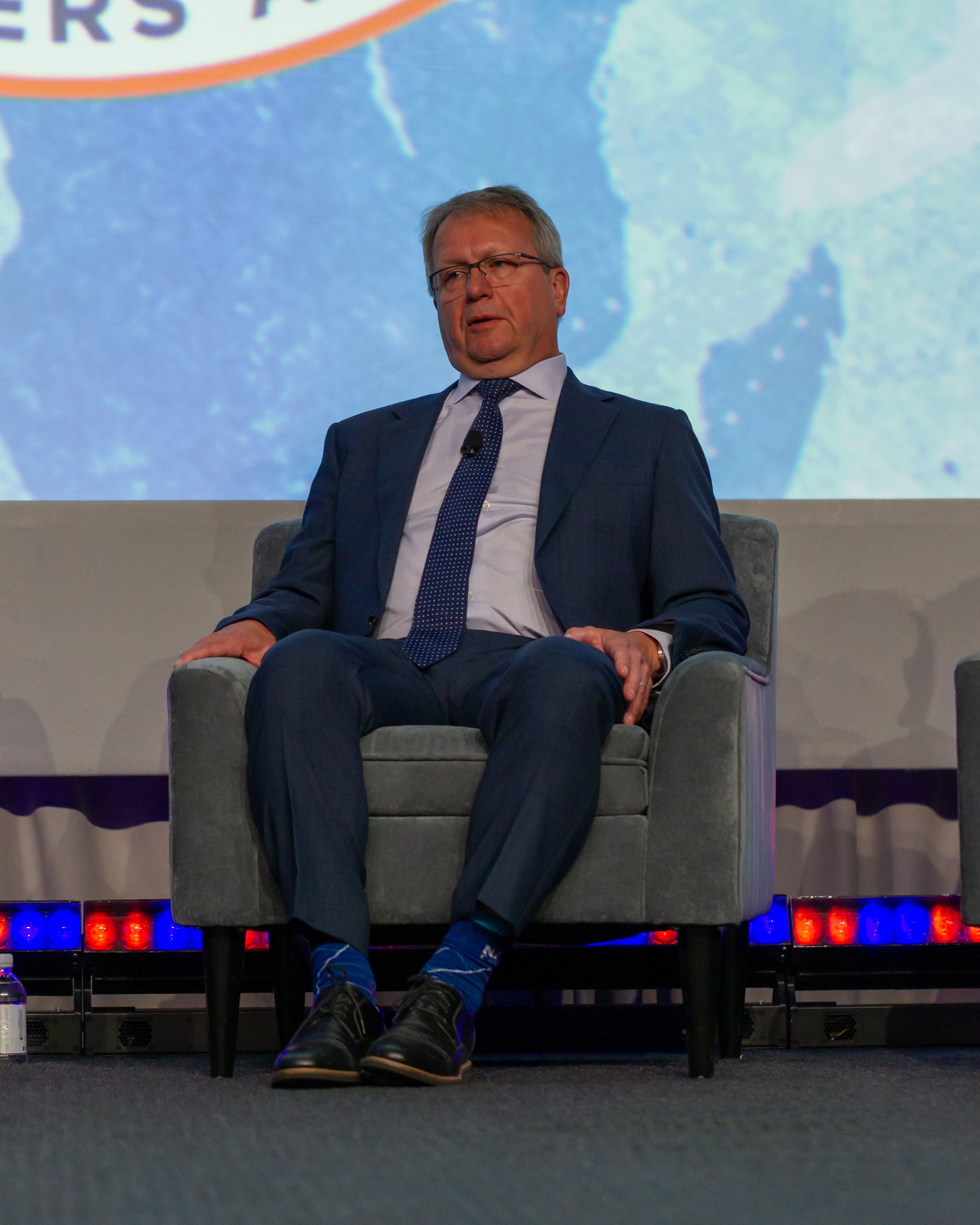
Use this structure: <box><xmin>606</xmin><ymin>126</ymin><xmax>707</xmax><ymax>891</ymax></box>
<box><xmin>0</xmin><ymin>953</ymin><xmax>28</xmax><ymax>1069</ymax></box>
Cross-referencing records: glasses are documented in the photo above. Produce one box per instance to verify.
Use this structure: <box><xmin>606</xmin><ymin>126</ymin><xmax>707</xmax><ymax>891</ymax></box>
<box><xmin>427</xmin><ymin>251</ymin><xmax>555</xmax><ymax>294</ymax></box>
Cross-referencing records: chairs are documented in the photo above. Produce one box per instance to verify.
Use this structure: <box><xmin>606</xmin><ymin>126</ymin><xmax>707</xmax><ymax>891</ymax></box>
<box><xmin>167</xmin><ymin>511</ymin><xmax>774</xmax><ymax>1076</ymax></box>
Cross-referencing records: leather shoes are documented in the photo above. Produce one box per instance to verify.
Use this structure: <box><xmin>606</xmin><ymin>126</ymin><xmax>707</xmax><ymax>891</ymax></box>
<box><xmin>360</xmin><ymin>978</ymin><xmax>476</xmax><ymax>1080</ymax></box>
<box><xmin>269</xmin><ymin>967</ymin><xmax>382</xmax><ymax>1084</ymax></box>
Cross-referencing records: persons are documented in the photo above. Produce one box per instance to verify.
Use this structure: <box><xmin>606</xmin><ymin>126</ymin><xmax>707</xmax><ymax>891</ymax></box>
<box><xmin>178</xmin><ymin>182</ymin><xmax>753</xmax><ymax>1088</ymax></box>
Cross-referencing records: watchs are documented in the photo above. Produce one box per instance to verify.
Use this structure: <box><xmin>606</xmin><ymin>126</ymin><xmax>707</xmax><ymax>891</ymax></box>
<box><xmin>651</xmin><ymin>642</ymin><xmax>669</xmax><ymax>681</ymax></box>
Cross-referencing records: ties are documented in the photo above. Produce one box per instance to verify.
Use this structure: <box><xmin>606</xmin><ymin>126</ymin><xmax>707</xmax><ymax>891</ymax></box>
<box><xmin>400</xmin><ymin>379</ymin><xmax>525</xmax><ymax>670</ymax></box>
